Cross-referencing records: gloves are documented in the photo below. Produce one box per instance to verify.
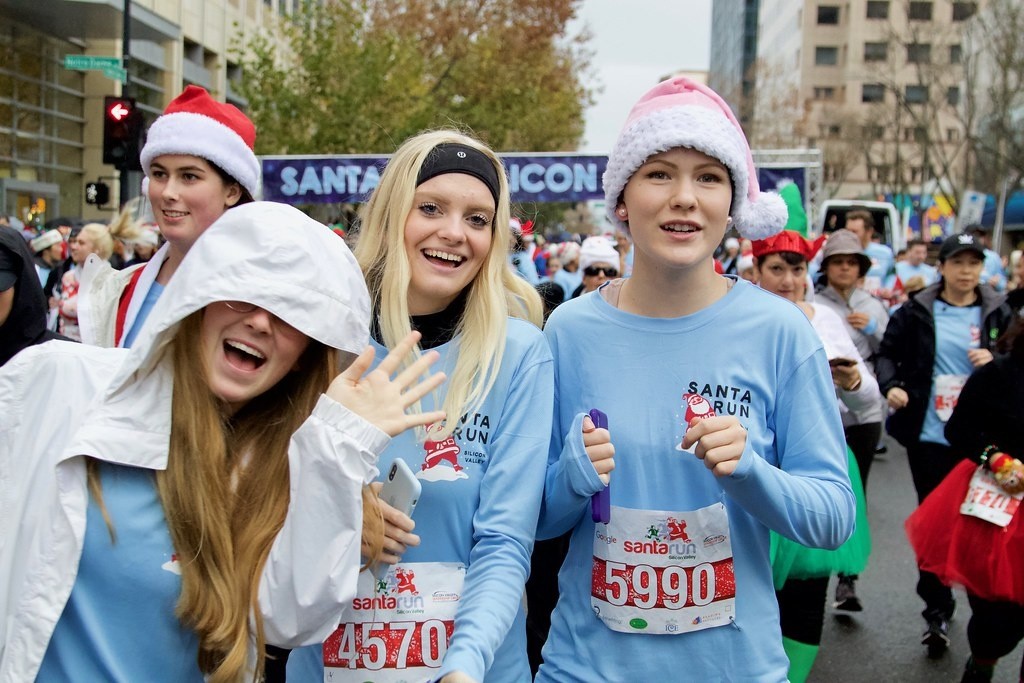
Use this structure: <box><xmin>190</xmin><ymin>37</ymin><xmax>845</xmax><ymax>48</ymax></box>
<box><xmin>989</xmin><ymin>453</ymin><xmax>1024</xmax><ymax>498</ymax></box>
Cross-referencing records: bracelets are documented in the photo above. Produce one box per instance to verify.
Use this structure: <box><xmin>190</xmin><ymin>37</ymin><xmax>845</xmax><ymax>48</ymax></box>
<box><xmin>981</xmin><ymin>444</ymin><xmax>999</xmax><ymax>463</ymax></box>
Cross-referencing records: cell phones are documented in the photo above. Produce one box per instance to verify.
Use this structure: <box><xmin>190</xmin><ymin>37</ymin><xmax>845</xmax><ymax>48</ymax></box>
<box><xmin>368</xmin><ymin>457</ymin><xmax>422</xmax><ymax>579</ymax></box>
<box><xmin>829</xmin><ymin>356</ymin><xmax>858</xmax><ymax>368</ymax></box>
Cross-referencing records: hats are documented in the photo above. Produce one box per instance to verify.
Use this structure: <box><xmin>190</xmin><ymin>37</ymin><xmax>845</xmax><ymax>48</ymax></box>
<box><xmin>520</xmin><ymin>220</ymin><xmax>535</xmax><ymax>242</ymax></box>
<box><xmin>816</xmin><ymin>228</ymin><xmax>871</xmax><ymax>278</ymax></box>
<box><xmin>25</xmin><ymin>220</ymin><xmax>63</xmax><ymax>254</ymax></box>
<box><xmin>0</xmin><ymin>243</ymin><xmax>25</xmax><ymax>291</ymax></box>
<box><xmin>581</xmin><ymin>237</ymin><xmax>620</xmax><ymax>272</ymax></box>
<box><xmin>509</xmin><ymin>216</ymin><xmax>522</xmax><ymax>235</ymax></box>
<box><xmin>129</xmin><ymin>219</ymin><xmax>161</xmax><ymax>245</ymax></box>
<box><xmin>601</xmin><ymin>75</ymin><xmax>789</xmax><ymax>242</ymax></box>
<box><xmin>140</xmin><ymin>83</ymin><xmax>259</xmax><ymax>196</ymax></box>
<box><xmin>939</xmin><ymin>235</ymin><xmax>985</xmax><ymax>262</ymax></box>
<box><xmin>549</xmin><ymin>241</ymin><xmax>580</xmax><ymax>266</ymax></box>
<box><xmin>964</xmin><ymin>223</ymin><xmax>988</xmax><ymax>235</ymax></box>
<box><xmin>752</xmin><ymin>177</ymin><xmax>827</xmax><ymax>262</ymax></box>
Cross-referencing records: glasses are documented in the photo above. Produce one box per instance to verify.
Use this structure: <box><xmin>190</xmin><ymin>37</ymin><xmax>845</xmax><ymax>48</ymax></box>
<box><xmin>827</xmin><ymin>258</ymin><xmax>860</xmax><ymax>266</ymax></box>
<box><xmin>583</xmin><ymin>265</ymin><xmax>618</xmax><ymax>278</ymax></box>
<box><xmin>223</xmin><ymin>300</ymin><xmax>302</xmax><ymax>336</ymax></box>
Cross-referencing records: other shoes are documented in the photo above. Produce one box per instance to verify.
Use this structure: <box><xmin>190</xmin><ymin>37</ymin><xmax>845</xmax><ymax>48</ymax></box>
<box><xmin>874</xmin><ymin>446</ymin><xmax>887</xmax><ymax>455</ymax></box>
<box><xmin>921</xmin><ymin>616</ymin><xmax>951</xmax><ymax>648</ymax></box>
<box><xmin>834</xmin><ymin>582</ymin><xmax>865</xmax><ymax>611</ymax></box>
<box><xmin>960</xmin><ymin>655</ymin><xmax>995</xmax><ymax>683</ymax></box>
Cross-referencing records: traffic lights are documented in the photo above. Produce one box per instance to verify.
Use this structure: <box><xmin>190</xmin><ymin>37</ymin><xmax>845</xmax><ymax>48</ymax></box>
<box><xmin>83</xmin><ymin>182</ymin><xmax>108</xmax><ymax>205</ymax></box>
<box><xmin>101</xmin><ymin>97</ymin><xmax>136</xmax><ymax>166</ymax></box>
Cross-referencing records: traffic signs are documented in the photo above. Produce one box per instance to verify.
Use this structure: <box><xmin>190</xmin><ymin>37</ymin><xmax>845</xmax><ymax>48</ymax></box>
<box><xmin>102</xmin><ymin>63</ymin><xmax>128</xmax><ymax>85</ymax></box>
<box><xmin>62</xmin><ymin>54</ymin><xmax>124</xmax><ymax>73</ymax></box>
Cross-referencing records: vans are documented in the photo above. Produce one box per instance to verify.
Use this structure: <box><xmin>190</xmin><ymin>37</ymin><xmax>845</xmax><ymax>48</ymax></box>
<box><xmin>814</xmin><ymin>198</ymin><xmax>903</xmax><ymax>256</ymax></box>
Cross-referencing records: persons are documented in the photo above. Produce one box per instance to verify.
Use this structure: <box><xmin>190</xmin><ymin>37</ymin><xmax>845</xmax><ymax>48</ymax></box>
<box><xmin>1</xmin><ymin>203</ymin><xmax>447</xmax><ymax>683</ymax></box>
<box><xmin>815</xmin><ymin>230</ymin><xmax>890</xmax><ymax>615</ymax></box>
<box><xmin>1</xmin><ymin>196</ymin><xmax>1022</xmax><ymax>457</ymax></box>
<box><xmin>2</xmin><ymin>223</ymin><xmax>50</xmax><ymax>361</ymax></box>
<box><xmin>747</xmin><ymin>226</ymin><xmax>871</xmax><ymax>682</ymax></box>
<box><xmin>944</xmin><ymin>307</ymin><xmax>1024</xmax><ymax>682</ymax></box>
<box><xmin>879</xmin><ymin>236</ymin><xmax>1016</xmax><ymax>653</ymax></box>
<box><xmin>77</xmin><ymin>87</ymin><xmax>263</xmax><ymax>348</ymax></box>
<box><xmin>282</xmin><ymin>127</ymin><xmax>554</xmax><ymax>683</ymax></box>
<box><xmin>530</xmin><ymin>75</ymin><xmax>857</xmax><ymax>683</ymax></box>
